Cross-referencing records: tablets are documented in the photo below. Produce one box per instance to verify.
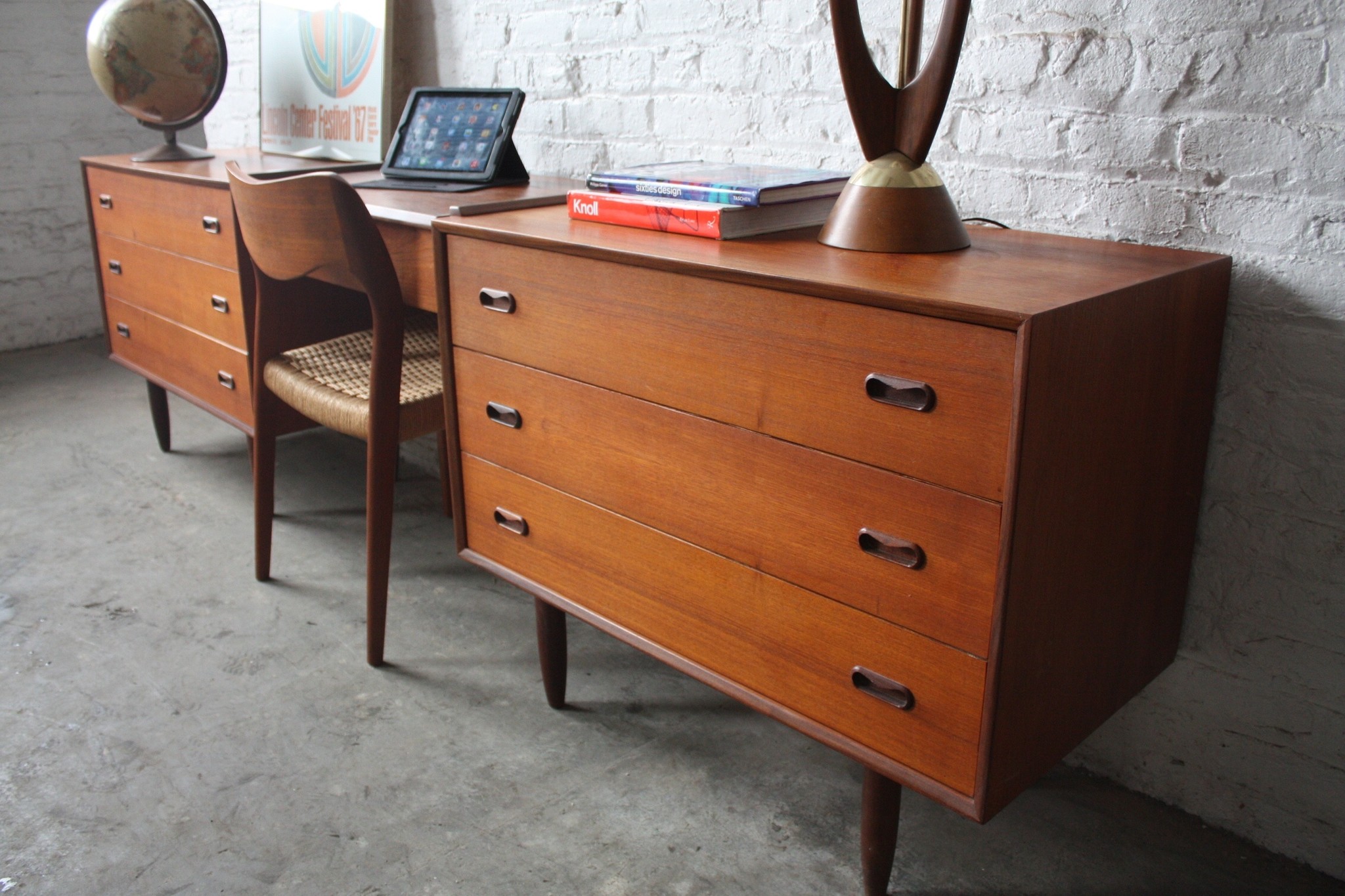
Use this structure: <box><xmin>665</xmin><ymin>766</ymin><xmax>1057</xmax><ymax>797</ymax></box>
<box><xmin>380</xmin><ymin>87</ymin><xmax>525</xmax><ymax>182</ymax></box>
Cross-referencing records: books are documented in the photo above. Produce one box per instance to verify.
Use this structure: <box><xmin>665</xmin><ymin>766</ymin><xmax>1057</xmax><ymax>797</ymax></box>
<box><xmin>566</xmin><ymin>188</ymin><xmax>839</xmax><ymax>240</ymax></box>
<box><xmin>585</xmin><ymin>160</ymin><xmax>854</xmax><ymax>206</ymax></box>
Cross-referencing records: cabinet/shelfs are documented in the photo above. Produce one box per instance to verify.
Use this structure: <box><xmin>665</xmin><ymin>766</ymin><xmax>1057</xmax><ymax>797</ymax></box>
<box><xmin>77</xmin><ymin>145</ymin><xmax>1238</xmax><ymax>896</ymax></box>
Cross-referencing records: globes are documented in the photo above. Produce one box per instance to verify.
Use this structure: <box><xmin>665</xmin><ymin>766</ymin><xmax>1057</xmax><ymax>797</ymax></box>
<box><xmin>86</xmin><ymin>0</ymin><xmax>228</xmax><ymax>162</ymax></box>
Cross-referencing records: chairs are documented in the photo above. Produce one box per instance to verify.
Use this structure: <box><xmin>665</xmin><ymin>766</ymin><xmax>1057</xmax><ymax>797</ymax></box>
<box><xmin>222</xmin><ymin>159</ymin><xmax>470</xmax><ymax>668</ymax></box>
